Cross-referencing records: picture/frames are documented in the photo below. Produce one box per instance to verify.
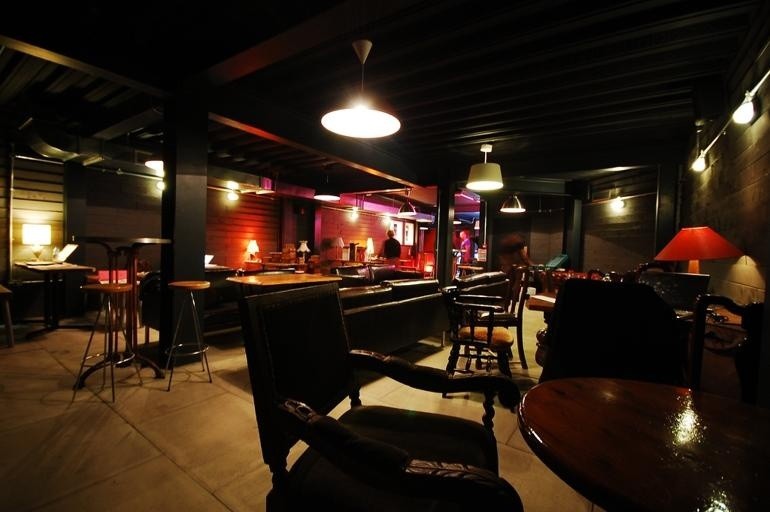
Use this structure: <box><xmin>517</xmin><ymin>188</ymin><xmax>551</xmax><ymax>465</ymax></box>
<box><xmin>390</xmin><ymin>221</ymin><xmax>404</xmax><ymax>246</ymax></box>
<box><xmin>404</xmin><ymin>222</ymin><xmax>415</xmax><ymax>246</ymax></box>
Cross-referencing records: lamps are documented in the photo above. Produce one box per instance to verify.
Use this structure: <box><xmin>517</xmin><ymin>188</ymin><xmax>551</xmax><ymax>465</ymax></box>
<box><xmin>310</xmin><ymin>176</ymin><xmax>341</xmax><ymax>202</ymax></box>
<box><xmin>463</xmin><ymin>143</ymin><xmax>505</xmax><ymax>191</ymax></box>
<box><xmin>397</xmin><ymin>187</ymin><xmax>418</xmax><ymax>219</ymax></box>
<box><xmin>452</xmin><ymin>213</ymin><xmax>462</xmax><ymax>225</ymax></box>
<box><xmin>21</xmin><ymin>220</ymin><xmax>54</xmax><ymax>262</ymax></box>
<box><xmin>654</xmin><ymin>225</ymin><xmax>747</xmax><ymax>273</ymax></box>
<box><xmin>498</xmin><ymin>191</ymin><xmax>526</xmax><ymax>216</ymax></box>
<box><xmin>419</xmin><ymin>223</ymin><xmax>430</xmax><ymax>230</ymax></box>
<box><xmin>318</xmin><ymin>38</ymin><xmax>400</xmax><ymax>140</ymax></box>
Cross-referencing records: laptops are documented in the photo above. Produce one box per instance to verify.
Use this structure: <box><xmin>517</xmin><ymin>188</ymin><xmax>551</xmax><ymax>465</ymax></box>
<box><xmin>640</xmin><ymin>271</ymin><xmax>711</xmax><ymax>312</ymax></box>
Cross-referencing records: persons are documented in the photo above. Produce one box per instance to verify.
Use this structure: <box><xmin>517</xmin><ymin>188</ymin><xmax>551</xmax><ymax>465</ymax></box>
<box><xmin>454</xmin><ymin>231</ymin><xmax>472</xmax><ymax>264</ymax></box>
<box><xmin>378</xmin><ymin>230</ymin><xmax>401</xmax><ymax>270</ymax></box>
